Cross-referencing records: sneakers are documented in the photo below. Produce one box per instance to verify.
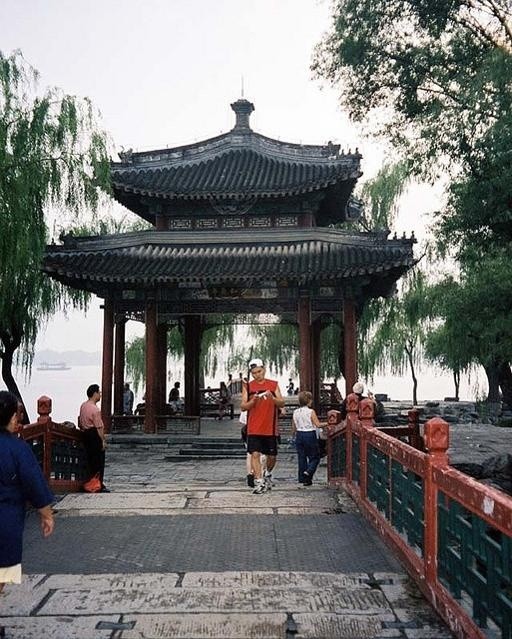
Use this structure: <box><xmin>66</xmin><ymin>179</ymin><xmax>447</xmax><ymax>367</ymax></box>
<box><xmin>253</xmin><ymin>479</ymin><xmax>266</xmax><ymax>494</ymax></box>
<box><xmin>262</xmin><ymin>470</ymin><xmax>275</xmax><ymax>491</ymax></box>
<box><xmin>247</xmin><ymin>472</ymin><xmax>254</xmax><ymax>488</ymax></box>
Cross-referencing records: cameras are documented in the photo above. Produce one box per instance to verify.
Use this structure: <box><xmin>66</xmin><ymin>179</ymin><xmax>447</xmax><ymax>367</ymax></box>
<box><xmin>258</xmin><ymin>392</ymin><xmax>268</xmax><ymax>400</ymax></box>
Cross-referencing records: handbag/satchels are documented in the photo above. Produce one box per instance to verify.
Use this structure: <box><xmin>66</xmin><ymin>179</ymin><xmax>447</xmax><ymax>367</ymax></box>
<box><xmin>77</xmin><ymin>472</ymin><xmax>102</xmax><ymax>493</ymax></box>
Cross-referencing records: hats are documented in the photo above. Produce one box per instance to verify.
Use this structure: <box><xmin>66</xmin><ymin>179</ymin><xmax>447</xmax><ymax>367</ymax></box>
<box><xmin>249</xmin><ymin>358</ymin><xmax>265</xmax><ymax>370</ymax></box>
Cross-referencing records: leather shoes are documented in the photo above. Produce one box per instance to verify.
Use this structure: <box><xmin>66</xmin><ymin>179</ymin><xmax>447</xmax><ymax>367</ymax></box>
<box><xmin>101</xmin><ymin>487</ymin><xmax>110</xmax><ymax>493</ymax></box>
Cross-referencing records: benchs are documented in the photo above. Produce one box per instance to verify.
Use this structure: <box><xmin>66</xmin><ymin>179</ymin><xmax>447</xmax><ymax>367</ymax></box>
<box><xmin>278</xmin><ymin>404</ymin><xmax>346</xmax><ymax>421</ymax></box>
<box><xmin>199</xmin><ymin>402</ymin><xmax>235</xmax><ymax>421</ymax></box>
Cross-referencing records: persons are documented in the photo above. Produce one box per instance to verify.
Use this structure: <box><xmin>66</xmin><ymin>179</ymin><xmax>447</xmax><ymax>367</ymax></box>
<box><xmin>218</xmin><ymin>382</ymin><xmax>232</xmax><ymax>420</ymax></box>
<box><xmin>291</xmin><ymin>391</ymin><xmax>329</xmax><ymax>486</ymax></box>
<box><xmin>294</xmin><ymin>388</ymin><xmax>300</xmax><ymax>395</ymax></box>
<box><xmin>0</xmin><ymin>391</ymin><xmax>55</xmax><ymax>637</ymax></box>
<box><xmin>316</xmin><ymin>427</ymin><xmax>327</xmax><ymax>466</ymax></box>
<box><xmin>242</xmin><ymin>358</ymin><xmax>284</xmax><ymax>493</ymax></box>
<box><xmin>240</xmin><ymin>411</ymin><xmax>256</xmax><ymax>488</ymax></box>
<box><xmin>169</xmin><ymin>382</ymin><xmax>180</xmax><ymax>402</ymax></box>
<box><xmin>342</xmin><ymin>383</ymin><xmax>383</xmax><ymax>420</ymax></box>
<box><xmin>124</xmin><ymin>383</ymin><xmax>134</xmax><ymax>414</ymax></box>
<box><xmin>286</xmin><ymin>378</ymin><xmax>294</xmax><ymax>396</ymax></box>
<box><xmin>80</xmin><ymin>384</ymin><xmax>110</xmax><ymax>492</ymax></box>
